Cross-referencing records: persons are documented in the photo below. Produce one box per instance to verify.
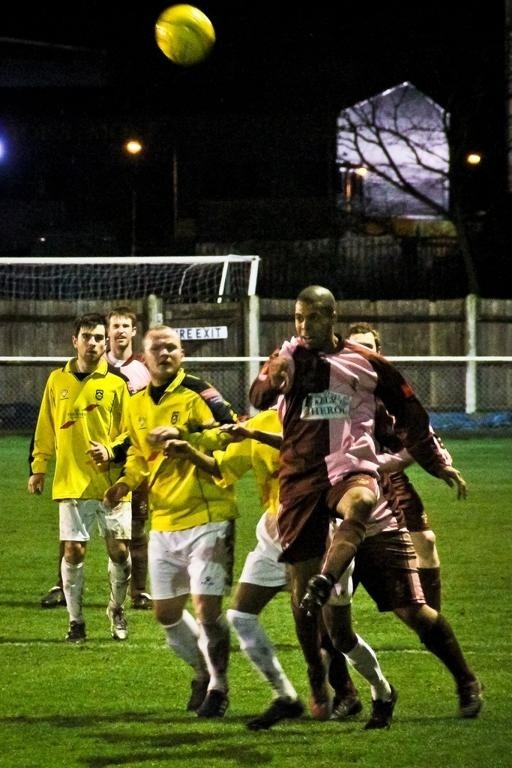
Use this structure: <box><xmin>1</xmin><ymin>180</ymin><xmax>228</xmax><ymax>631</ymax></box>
<box><xmin>27</xmin><ymin>307</ymin><xmax>149</xmax><ymax>641</ymax></box>
<box><xmin>164</xmin><ymin>287</ymin><xmax>484</xmax><ymax>732</ymax></box>
<box><xmin>100</xmin><ymin>326</ymin><xmax>242</xmax><ymax>717</ymax></box>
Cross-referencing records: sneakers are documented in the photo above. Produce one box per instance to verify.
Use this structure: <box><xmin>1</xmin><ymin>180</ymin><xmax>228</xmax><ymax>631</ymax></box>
<box><xmin>131</xmin><ymin>592</ymin><xmax>153</xmax><ymax>609</ymax></box>
<box><xmin>65</xmin><ymin>621</ymin><xmax>86</xmax><ymax>642</ymax></box>
<box><xmin>106</xmin><ymin>602</ymin><xmax>127</xmax><ymax>640</ymax></box>
<box><xmin>364</xmin><ymin>683</ymin><xmax>397</xmax><ymax>729</ymax></box>
<box><xmin>185</xmin><ymin>680</ymin><xmax>362</xmax><ymax>729</ymax></box>
<box><xmin>41</xmin><ymin>586</ymin><xmax>67</xmax><ymax>606</ymax></box>
<box><xmin>454</xmin><ymin>674</ymin><xmax>484</xmax><ymax>717</ymax></box>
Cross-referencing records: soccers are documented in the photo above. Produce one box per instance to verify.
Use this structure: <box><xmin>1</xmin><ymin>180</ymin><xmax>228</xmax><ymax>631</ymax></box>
<box><xmin>156</xmin><ymin>4</ymin><xmax>215</xmax><ymax>66</ymax></box>
